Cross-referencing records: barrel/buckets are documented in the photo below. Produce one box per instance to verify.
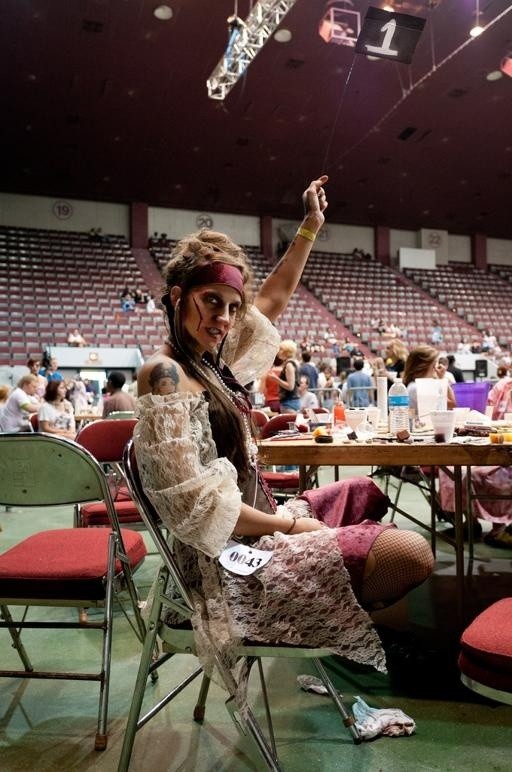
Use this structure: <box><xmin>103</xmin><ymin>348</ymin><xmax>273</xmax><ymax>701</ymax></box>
<box><xmin>452</xmin><ymin>381</ymin><xmax>495</xmax><ymax>416</ymax></box>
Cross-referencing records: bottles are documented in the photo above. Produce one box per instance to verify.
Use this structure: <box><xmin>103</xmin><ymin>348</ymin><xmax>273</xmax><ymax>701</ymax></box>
<box><xmin>388</xmin><ymin>376</ymin><xmax>408</xmax><ymax>435</ymax></box>
<box><xmin>334</xmin><ymin>402</ymin><xmax>344</xmax><ymax>428</ymax></box>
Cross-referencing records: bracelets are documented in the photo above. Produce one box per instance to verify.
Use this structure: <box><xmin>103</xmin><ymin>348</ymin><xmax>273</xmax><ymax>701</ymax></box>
<box><xmin>296</xmin><ymin>227</ymin><xmax>316</xmax><ymax>241</ymax></box>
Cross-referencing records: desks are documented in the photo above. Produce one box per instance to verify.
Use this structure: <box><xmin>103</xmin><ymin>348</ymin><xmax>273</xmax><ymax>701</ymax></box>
<box><xmin>244</xmin><ymin>420</ymin><xmax>512</xmax><ymax>714</ymax></box>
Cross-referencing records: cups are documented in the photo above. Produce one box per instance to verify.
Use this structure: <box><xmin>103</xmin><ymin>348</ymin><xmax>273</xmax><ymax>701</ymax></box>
<box><xmin>427</xmin><ymin>410</ymin><xmax>457</xmax><ymax>444</ymax></box>
<box><xmin>450</xmin><ymin>382</ymin><xmax>494</xmax><ymax>418</ymax></box>
<box><xmin>343</xmin><ymin>407</ymin><xmax>380</xmax><ymax>436</ymax></box>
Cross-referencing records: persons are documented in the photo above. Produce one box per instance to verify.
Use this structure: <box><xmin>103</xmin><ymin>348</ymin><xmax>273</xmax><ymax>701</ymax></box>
<box><xmin>149</xmin><ymin>232</ymin><xmax>168</xmax><ymax>246</ymax></box>
<box><xmin>89</xmin><ymin>228</ymin><xmax>105</xmax><ymax>242</ymax></box>
<box><xmin>134</xmin><ymin>175</ymin><xmax>433</xmax><ymax>676</ymax></box>
<box><xmin>121</xmin><ymin>286</ymin><xmax>152</xmax><ymax>311</ymax></box>
<box><xmin>353</xmin><ymin>248</ymin><xmax>371</xmax><ymax>260</ymax></box>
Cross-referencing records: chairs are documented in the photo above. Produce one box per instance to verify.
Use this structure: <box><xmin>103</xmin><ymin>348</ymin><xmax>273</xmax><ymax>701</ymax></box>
<box><xmin>61</xmin><ymin>396</ymin><xmax>345</xmax><ymax>622</ymax></box>
<box><xmin>452</xmin><ymin>592</ymin><xmax>511</xmax><ymax>713</ymax></box>
<box><xmin>103</xmin><ymin>431</ymin><xmax>371</xmax><ymax>770</ymax></box>
<box><xmin>0</xmin><ymin>216</ymin><xmax>512</xmax><ymax>408</ymax></box>
<box><xmin>0</xmin><ymin>429</ymin><xmax>161</xmax><ymax>757</ymax></box>
<box><xmin>377</xmin><ymin>463</ymin><xmax>439</xmax><ymax>525</ymax></box>
